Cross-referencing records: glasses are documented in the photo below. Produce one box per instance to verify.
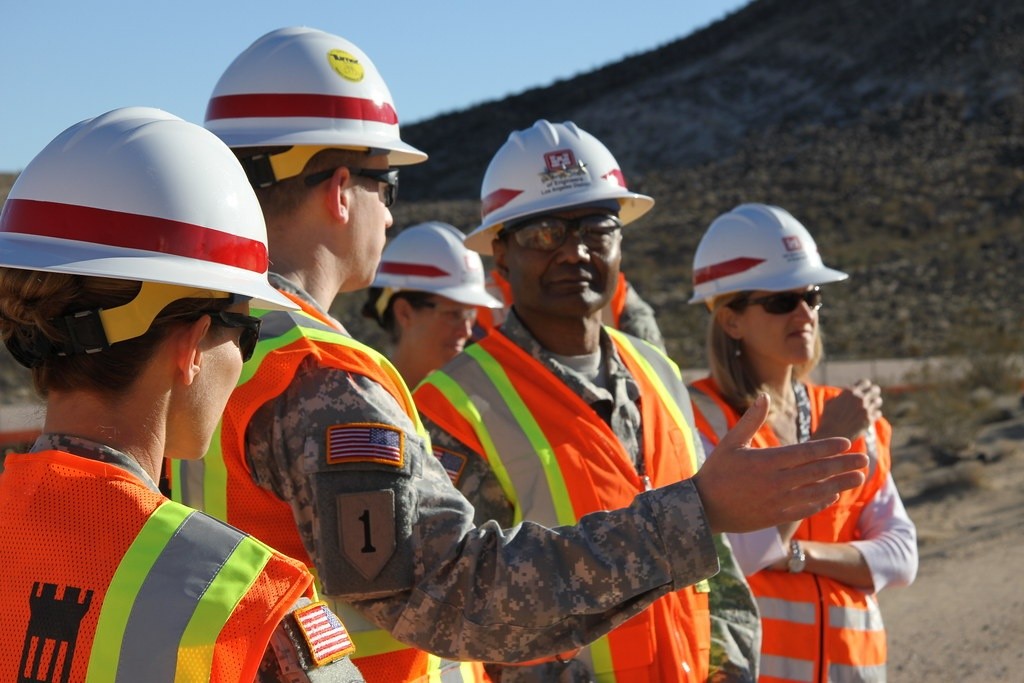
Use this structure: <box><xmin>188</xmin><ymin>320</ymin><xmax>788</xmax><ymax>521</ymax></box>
<box><xmin>724</xmin><ymin>286</ymin><xmax>824</xmax><ymax>314</ymax></box>
<box><xmin>497</xmin><ymin>212</ymin><xmax>624</xmax><ymax>252</ymax></box>
<box><xmin>152</xmin><ymin>308</ymin><xmax>261</xmax><ymax>362</ymax></box>
<box><xmin>304</xmin><ymin>167</ymin><xmax>399</xmax><ymax>207</ymax></box>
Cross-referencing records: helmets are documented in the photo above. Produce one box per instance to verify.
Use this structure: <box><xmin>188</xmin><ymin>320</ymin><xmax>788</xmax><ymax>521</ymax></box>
<box><xmin>368</xmin><ymin>221</ymin><xmax>503</xmax><ymax>308</ymax></box>
<box><xmin>204</xmin><ymin>25</ymin><xmax>428</xmax><ymax>165</ymax></box>
<box><xmin>464</xmin><ymin>119</ymin><xmax>655</xmax><ymax>257</ymax></box>
<box><xmin>0</xmin><ymin>107</ymin><xmax>302</xmax><ymax>312</ymax></box>
<box><xmin>687</xmin><ymin>202</ymin><xmax>848</xmax><ymax>302</ymax></box>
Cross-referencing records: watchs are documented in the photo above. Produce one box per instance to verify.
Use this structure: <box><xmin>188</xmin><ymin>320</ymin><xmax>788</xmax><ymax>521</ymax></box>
<box><xmin>789</xmin><ymin>540</ymin><xmax>805</xmax><ymax>574</ymax></box>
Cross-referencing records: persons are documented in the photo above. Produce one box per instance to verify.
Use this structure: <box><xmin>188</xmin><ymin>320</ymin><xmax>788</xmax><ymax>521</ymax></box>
<box><xmin>684</xmin><ymin>201</ymin><xmax>918</xmax><ymax>683</ymax></box>
<box><xmin>1</xmin><ymin>24</ymin><xmax>870</xmax><ymax>683</ymax></box>
